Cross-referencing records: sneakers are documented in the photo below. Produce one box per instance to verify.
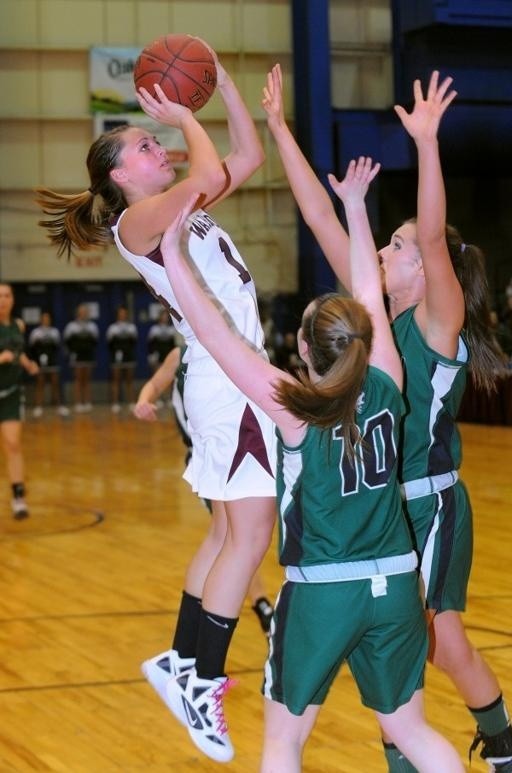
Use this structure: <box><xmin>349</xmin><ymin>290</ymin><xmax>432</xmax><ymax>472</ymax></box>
<box><xmin>468</xmin><ymin>725</ymin><xmax>512</xmax><ymax>773</ymax></box>
<box><xmin>11</xmin><ymin>497</ymin><xmax>28</xmax><ymax>519</ymax></box>
<box><xmin>141</xmin><ymin>649</ymin><xmax>196</xmax><ymax>728</ymax></box>
<box><xmin>166</xmin><ymin>667</ymin><xmax>239</xmax><ymax>763</ymax></box>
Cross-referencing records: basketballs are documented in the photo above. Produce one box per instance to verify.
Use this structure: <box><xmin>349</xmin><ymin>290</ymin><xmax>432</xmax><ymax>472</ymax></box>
<box><xmin>134</xmin><ymin>31</ymin><xmax>220</xmax><ymax>112</ymax></box>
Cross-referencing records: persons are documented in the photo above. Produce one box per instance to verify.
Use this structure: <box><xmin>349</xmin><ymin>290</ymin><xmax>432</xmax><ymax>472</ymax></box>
<box><xmin>146</xmin><ymin>311</ymin><xmax>172</xmax><ymax>371</ymax></box>
<box><xmin>107</xmin><ymin>310</ymin><xmax>136</xmax><ymax>414</ymax></box>
<box><xmin>65</xmin><ymin>305</ymin><xmax>100</xmax><ymax>413</ymax></box>
<box><xmin>160</xmin><ymin>157</ymin><xmax>467</xmax><ymax>773</ymax></box>
<box><xmin>30</xmin><ymin>312</ymin><xmax>68</xmax><ymax>419</ymax></box>
<box><xmin>263</xmin><ymin>62</ymin><xmax>512</xmax><ymax>773</ymax></box>
<box><xmin>37</xmin><ymin>36</ymin><xmax>278</xmax><ymax>762</ymax></box>
<box><xmin>0</xmin><ymin>281</ymin><xmax>37</xmax><ymax>521</ymax></box>
